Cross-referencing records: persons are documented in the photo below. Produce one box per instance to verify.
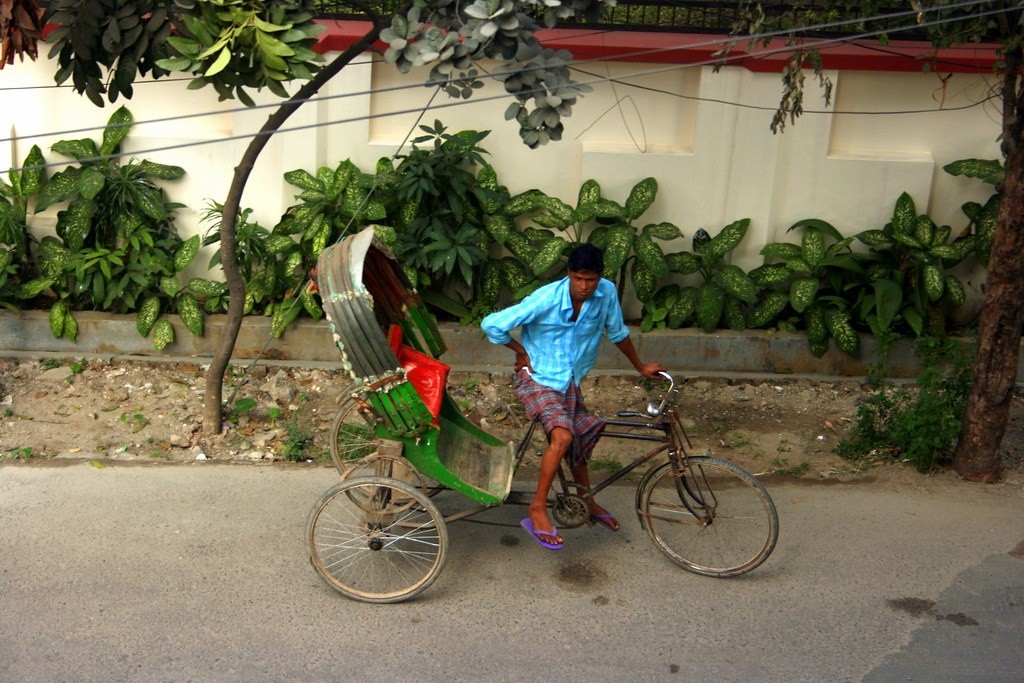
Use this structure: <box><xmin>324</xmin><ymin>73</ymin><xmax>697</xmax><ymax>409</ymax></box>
<box><xmin>480</xmin><ymin>244</ymin><xmax>667</xmax><ymax>550</ymax></box>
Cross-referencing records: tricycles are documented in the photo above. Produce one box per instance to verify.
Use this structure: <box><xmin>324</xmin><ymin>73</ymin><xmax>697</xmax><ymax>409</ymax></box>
<box><xmin>304</xmin><ymin>223</ymin><xmax>778</xmax><ymax>605</ymax></box>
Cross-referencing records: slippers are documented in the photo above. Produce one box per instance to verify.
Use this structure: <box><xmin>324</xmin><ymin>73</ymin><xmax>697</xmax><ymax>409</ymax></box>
<box><xmin>590</xmin><ymin>507</ymin><xmax>620</xmax><ymax>532</ymax></box>
<box><xmin>520</xmin><ymin>518</ymin><xmax>564</xmax><ymax>550</ymax></box>
